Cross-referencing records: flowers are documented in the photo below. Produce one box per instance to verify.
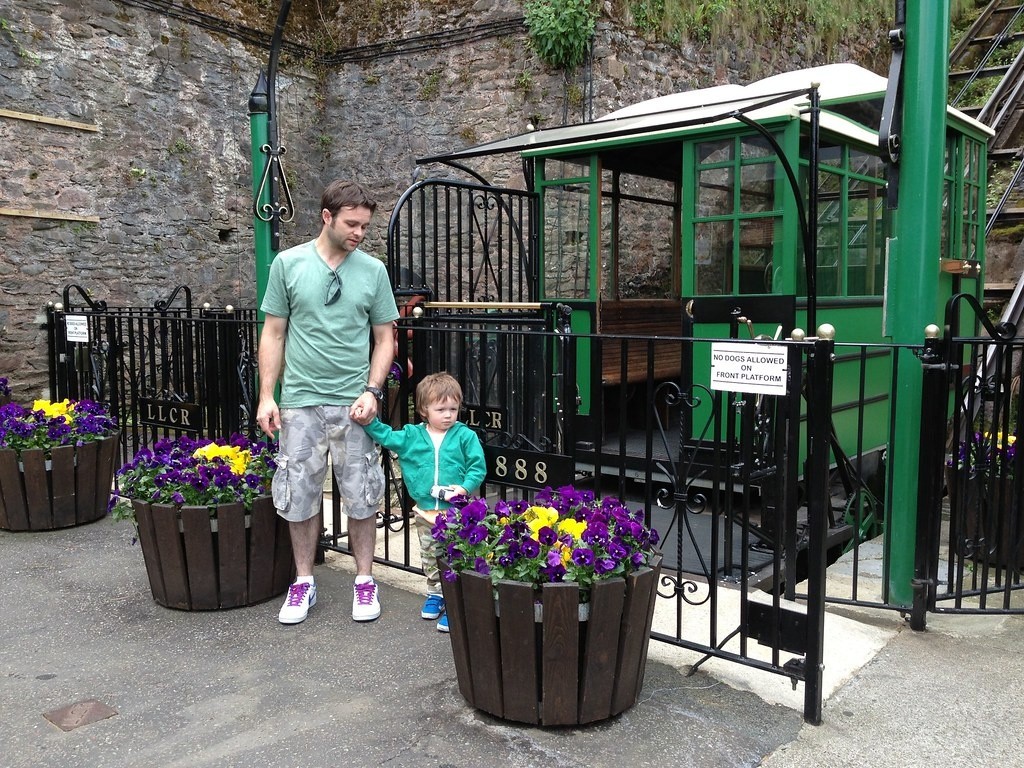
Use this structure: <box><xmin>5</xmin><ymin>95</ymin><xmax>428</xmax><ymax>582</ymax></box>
<box><xmin>949</xmin><ymin>428</ymin><xmax>1018</xmax><ymax>476</ymax></box>
<box><xmin>433</xmin><ymin>485</ymin><xmax>661</xmax><ymax>603</ymax></box>
<box><xmin>0</xmin><ymin>376</ymin><xmax>119</xmax><ymax>458</ymax></box>
<box><xmin>105</xmin><ymin>433</ymin><xmax>283</xmax><ymax>548</ymax></box>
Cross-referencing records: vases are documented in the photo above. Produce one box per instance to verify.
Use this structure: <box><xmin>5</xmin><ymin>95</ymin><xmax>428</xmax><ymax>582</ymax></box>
<box><xmin>127</xmin><ymin>493</ymin><xmax>296</xmax><ymax>613</ymax></box>
<box><xmin>948</xmin><ymin>471</ymin><xmax>1023</xmax><ymax>572</ymax></box>
<box><xmin>432</xmin><ymin>547</ymin><xmax>664</xmax><ymax>728</ymax></box>
<box><xmin>0</xmin><ymin>431</ymin><xmax>121</xmax><ymax>533</ymax></box>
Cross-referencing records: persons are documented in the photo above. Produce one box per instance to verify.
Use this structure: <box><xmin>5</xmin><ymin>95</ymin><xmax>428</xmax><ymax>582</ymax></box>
<box><xmin>256</xmin><ymin>180</ymin><xmax>400</xmax><ymax>624</ymax></box>
<box><xmin>350</xmin><ymin>372</ymin><xmax>487</xmax><ymax>631</ymax></box>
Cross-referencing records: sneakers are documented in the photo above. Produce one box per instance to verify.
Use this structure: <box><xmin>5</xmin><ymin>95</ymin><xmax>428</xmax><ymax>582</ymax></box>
<box><xmin>422</xmin><ymin>593</ymin><xmax>444</xmax><ymax>620</ymax></box>
<box><xmin>437</xmin><ymin>611</ymin><xmax>449</xmax><ymax>633</ymax></box>
<box><xmin>352</xmin><ymin>581</ymin><xmax>381</xmax><ymax>621</ymax></box>
<box><xmin>279</xmin><ymin>582</ymin><xmax>317</xmax><ymax>624</ymax></box>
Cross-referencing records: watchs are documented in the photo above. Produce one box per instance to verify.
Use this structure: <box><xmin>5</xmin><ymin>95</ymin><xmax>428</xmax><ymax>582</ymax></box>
<box><xmin>366</xmin><ymin>387</ymin><xmax>384</xmax><ymax>401</ymax></box>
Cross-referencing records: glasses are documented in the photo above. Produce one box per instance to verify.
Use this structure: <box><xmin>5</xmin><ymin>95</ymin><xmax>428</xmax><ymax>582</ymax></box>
<box><xmin>325</xmin><ymin>270</ymin><xmax>342</xmax><ymax>307</ymax></box>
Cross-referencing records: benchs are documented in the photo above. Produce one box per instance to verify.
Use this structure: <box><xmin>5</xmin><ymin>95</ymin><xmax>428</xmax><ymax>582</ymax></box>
<box><xmin>603</xmin><ymin>321</ymin><xmax>684</xmax><ymax>430</ymax></box>
